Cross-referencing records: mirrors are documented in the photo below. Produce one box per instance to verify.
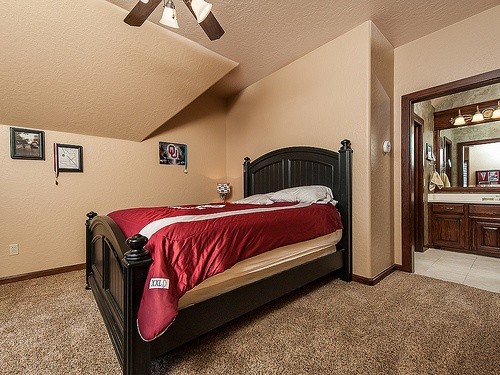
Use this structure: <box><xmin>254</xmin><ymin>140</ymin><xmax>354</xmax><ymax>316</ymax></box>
<box><xmin>434</xmin><ymin>99</ymin><xmax>500</xmax><ymax>193</ymax></box>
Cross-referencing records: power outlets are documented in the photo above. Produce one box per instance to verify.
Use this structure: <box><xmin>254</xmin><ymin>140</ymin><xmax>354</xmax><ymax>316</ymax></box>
<box><xmin>9</xmin><ymin>243</ymin><xmax>18</xmax><ymax>256</ymax></box>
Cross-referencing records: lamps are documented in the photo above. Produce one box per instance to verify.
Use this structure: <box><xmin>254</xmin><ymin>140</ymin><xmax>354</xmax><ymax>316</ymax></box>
<box><xmin>448</xmin><ymin>101</ymin><xmax>500</xmax><ymax>126</ymax></box>
<box><xmin>141</xmin><ymin>0</ymin><xmax>212</xmax><ymax>28</ymax></box>
<box><xmin>217</xmin><ymin>184</ymin><xmax>231</xmax><ymax>202</ymax></box>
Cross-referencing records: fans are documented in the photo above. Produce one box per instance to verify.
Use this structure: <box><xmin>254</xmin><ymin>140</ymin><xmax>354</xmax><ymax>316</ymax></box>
<box><xmin>122</xmin><ymin>0</ymin><xmax>225</xmax><ymax>41</ymax></box>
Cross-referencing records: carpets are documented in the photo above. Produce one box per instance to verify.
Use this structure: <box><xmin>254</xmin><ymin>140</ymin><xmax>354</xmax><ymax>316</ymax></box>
<box><xmin>0</xmin><ymin>270</ymin><xmax>500</xmax><ymax>375</ymax></box>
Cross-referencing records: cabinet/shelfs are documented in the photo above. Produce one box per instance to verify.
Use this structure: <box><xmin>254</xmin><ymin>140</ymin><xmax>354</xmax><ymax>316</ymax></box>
<box><xmin>428</xmin><ymin>203</ymin><xmax>500</xmax><ymax>258</ymax></box>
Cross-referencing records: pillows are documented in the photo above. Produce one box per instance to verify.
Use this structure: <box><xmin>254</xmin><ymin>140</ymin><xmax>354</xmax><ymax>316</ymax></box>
<box><xmin>270</xmin><ymin>185</ymin><xmax>334</xmax><ymax>204</ymax></box>
<box><xmin>231</xmin><ymin>192</ymin><xmax>274</xmax><ymax>205</ymax></box>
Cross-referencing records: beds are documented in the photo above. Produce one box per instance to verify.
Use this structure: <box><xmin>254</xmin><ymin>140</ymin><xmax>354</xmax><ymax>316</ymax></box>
<box><xmin>84</xmin><ymin>140</ymin><xmax>353</xmax><ymax>375</ymax></box>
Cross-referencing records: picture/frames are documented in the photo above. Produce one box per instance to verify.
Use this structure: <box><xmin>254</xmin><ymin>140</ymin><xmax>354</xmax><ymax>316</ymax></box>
<box><xmin>54</xmin><ymin>143</ymin><xmax>84</xmax><ymax>173</ymax></box>
<box><xmin>10</xmin><ymin>127</ymin><xmax>45</xmax><ymax>160</ymax></box>
<box><xmin>426</xmin><ymin>143</ymin><xmax>433</xmax><ymax>160</ymax></box>
